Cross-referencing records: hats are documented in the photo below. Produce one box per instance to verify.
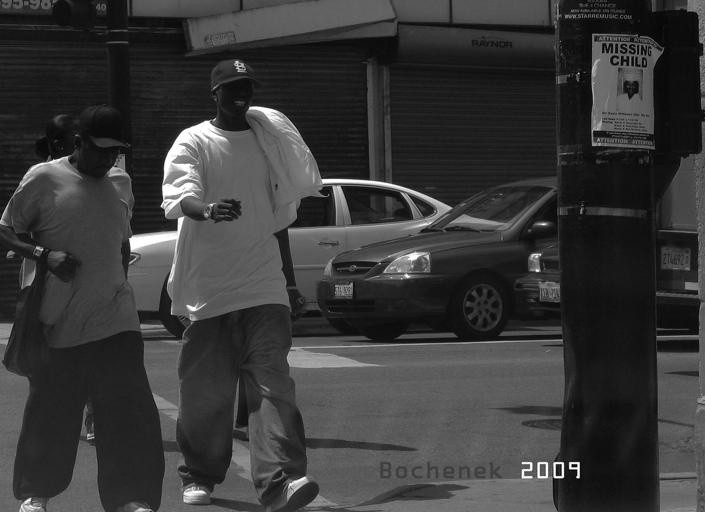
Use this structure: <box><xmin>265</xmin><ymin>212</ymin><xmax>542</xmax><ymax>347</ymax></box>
<box><xmin>76</xmin><ymin>103</ymin><xmax>130</xmax><ymax>150</ymax></box>
<box><xmin>210</xmin><ymin>58</ymin><xmax>256</xmax><ymax>91</ymax></box>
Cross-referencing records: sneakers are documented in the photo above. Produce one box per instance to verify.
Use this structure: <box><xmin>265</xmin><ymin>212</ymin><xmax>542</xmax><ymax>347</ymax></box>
<box><xmin>182</xmin><ymin>483</ymin><xmax>213</xmax><ymax>505</ymax></box>
<box><xmin>18</xmin><ymin>497</ymin><xmax>48</xmax><ymax>512</ymax></box>
<box><xmin>117</xmin><ymin>500</ymin><xmax>154</xmax><ymax>512</ymax></box>
<box><xmin>265</xmin><ymin>474</ymin><xmax>320</xmax><ymax>512</ymax></box>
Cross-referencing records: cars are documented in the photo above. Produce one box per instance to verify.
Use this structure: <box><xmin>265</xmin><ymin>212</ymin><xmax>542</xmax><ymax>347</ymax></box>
<box><xmin>125</xmin><ymin>179</ymin><xmax>507</xmax><ymax>339</ymax></box>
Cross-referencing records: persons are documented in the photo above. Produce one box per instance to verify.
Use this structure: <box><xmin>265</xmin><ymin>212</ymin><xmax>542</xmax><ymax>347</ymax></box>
<box><xmin>1</xmin><ymin>104</ymin><xmax>168</xmax><ymax>512</ymax></box>
<box><xmin>616</xmin><ymin>74</ymin><xmax>642</xmax><ymax>104</ymax></box>
<box><xmin>159</xmin><ymin>57</ymin><xmax>329</xmax><ymax>512</ymax></box>
<box><xmin>33</xmin><ymin>112</ymin><xmax>96</xmax><ymax>441</ymax></box>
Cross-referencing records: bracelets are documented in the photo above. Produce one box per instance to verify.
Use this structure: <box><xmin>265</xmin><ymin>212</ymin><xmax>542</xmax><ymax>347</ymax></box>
<box><xmin>286</xmin><ymin>285</ymin><xmax>299</xmax><ymax>290</ymax></box>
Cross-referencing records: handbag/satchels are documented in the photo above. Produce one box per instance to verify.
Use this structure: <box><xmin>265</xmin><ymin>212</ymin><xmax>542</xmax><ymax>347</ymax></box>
<box><xmin>2</xmin><ymin>246</ymin><xmax>52</xmax><ymax>376</ymax></box>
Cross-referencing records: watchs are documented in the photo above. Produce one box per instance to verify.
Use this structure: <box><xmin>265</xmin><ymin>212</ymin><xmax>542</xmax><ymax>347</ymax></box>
<box><xmin>204</xmin><ymin>201</ymin><xmax>216</xmax><ymax>222</ymax></box>
<box><xmin>32</xmin><ymin>243</ymin><xmax>45</xmax><ymax>260</ymax></box>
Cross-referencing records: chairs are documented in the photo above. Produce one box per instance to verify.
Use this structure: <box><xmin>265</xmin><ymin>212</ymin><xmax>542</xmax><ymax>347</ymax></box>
<box><xmin>393</xmin><ymin>209</ymin><xmax>408</xmax><ymax>219</ymax></box>
